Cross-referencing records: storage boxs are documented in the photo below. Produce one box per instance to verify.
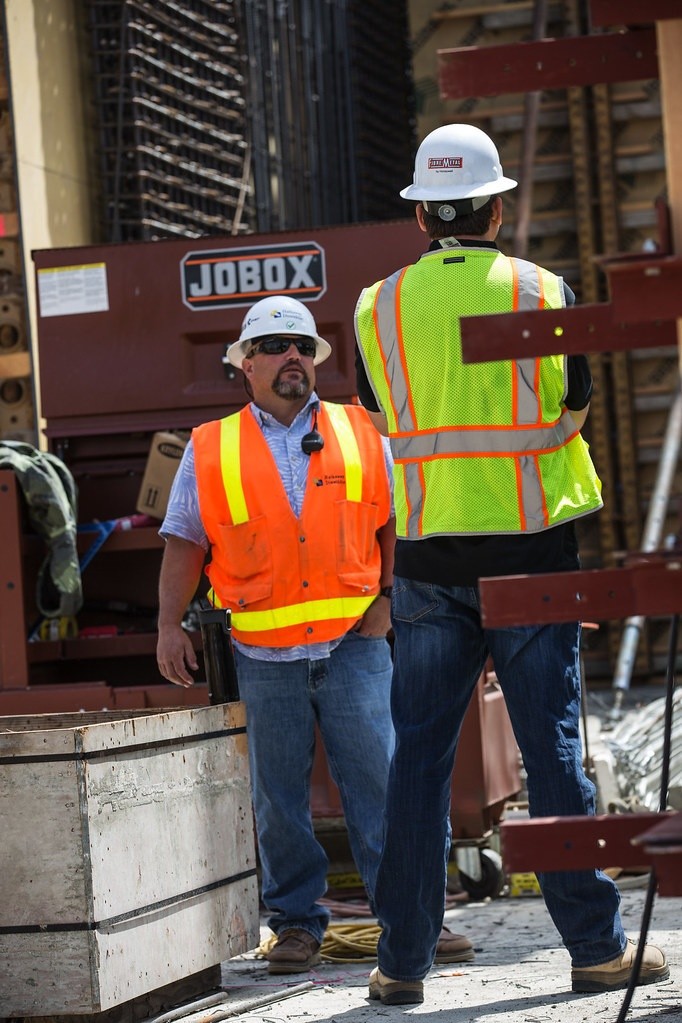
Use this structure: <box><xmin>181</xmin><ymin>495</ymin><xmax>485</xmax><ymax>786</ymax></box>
<box><xmin>135</xmin><ymin>431</ymin><xmax>191</xmax><ymax>520</ymax></box>
<box><xmin>0</xmin><ymin>701</ymin><xmax>259</xmax><ymax>1023</ymax></box>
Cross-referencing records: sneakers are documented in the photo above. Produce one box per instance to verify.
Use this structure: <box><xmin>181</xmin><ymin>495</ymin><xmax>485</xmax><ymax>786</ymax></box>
<box><xmin>268</xmin><ymin>928</ymin><xmax>321</xmax><ymax>973</ymax></box>
<box><xmin>369</xmin><ymin>967</ymin><xmax>424</xmax><ymax>1004</ymax></box>
<box><xmin>435</xmin><ymin>925</ymin><xmax>475</xmax><ymax>962</ymax></box>
<box><xmin>571</xmin><ymin>938</ymin><xmax>670</xmax><ymax>993</ymax></box>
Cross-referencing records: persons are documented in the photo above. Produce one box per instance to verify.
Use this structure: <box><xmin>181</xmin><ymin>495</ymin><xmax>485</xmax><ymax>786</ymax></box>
<box><xmin>349</xmin><ymin>124</ymin><xmax>672</xmax><ymax>1004</ymax></box>
<box><xmin>157</xmin><ymin>296</ymin><xmax>474</xmax><ymax>974</ymax></box>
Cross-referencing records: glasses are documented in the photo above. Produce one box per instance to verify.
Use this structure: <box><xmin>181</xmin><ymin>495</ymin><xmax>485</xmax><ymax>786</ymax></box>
<box><xmin>245</xmin><ymin>337</ymin><xmax>317</xmax><ymax>360</ymax></box>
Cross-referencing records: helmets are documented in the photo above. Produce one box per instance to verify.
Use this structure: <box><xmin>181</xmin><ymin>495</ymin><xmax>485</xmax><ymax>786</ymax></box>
<box><xmin>227</xmin><ymin>296</ymin><xmax>332</xmax><ymax>370</ymax></box>
<box><xmin>400</xmin><ymin>124</ymin><xmax>519</xmax><ymax>202</ymax></box>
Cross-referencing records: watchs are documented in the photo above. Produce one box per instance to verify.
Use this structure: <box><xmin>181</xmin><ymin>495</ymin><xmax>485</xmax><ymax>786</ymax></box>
<box><xmin>380</xmin><ymin>586</ymin><xmax>394</xmax><ymax>598</ymax></box>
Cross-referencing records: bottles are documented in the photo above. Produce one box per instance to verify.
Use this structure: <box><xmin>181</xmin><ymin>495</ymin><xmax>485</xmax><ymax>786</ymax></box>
<box><xmin>198</xmin><ymin>608</ymin><xmax>239</xmax><ymax>706</ymax></box>
